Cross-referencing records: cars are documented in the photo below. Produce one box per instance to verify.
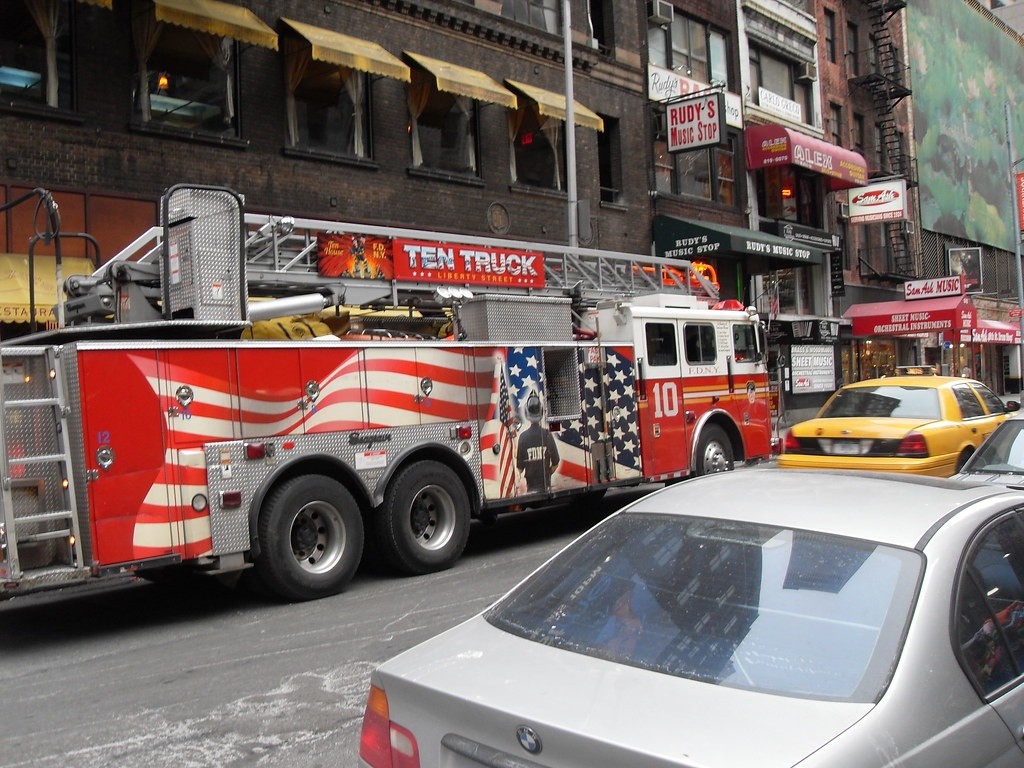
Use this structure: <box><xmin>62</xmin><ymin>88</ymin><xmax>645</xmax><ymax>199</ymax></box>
<box><xmin>359</xmin><ymin>462</ymin><xmax>1024</xmax><ymax>768</ymax></box>
<box><xmin>945</xmin><ymin>415</ymin><xmax>1024</xmax><ymax>484</ymax></box>
<box><xmin>774</xmin><ymin>365</ymin><xmax>1020</xmax><ymax>476</ymax></box>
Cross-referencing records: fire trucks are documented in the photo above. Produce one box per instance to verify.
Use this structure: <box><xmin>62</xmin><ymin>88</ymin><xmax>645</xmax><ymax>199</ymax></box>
<box><xmin>0</xmin><ymin>184</ymin><xmax>776</xmax><ymax>614</ymax></box>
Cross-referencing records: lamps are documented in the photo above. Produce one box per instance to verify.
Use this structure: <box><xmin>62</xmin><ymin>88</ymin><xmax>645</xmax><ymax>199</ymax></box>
<box><xmin>978</xmin><ymin>288</ymin><xmax>1015</xmax><ymax>296</ymax></box>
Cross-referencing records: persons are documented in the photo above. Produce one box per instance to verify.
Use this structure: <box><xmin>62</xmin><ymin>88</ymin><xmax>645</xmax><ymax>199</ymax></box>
<box><xmin>517</xmin><ymin>391</ymin><xmax>560</xmax><ymax>493</ymax></box>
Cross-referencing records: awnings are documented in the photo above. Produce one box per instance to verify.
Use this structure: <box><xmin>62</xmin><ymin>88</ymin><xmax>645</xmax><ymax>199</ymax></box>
<box><xmin>960</xmin><ymin>319</ymin><xmax>1021</xmax><ymax>344</ymax></box>
<box><xmin>280</xmin><ymin>17</ymin><xmax>411</xmax><ymax>84</ymax></box>
<box><xmin>746</xmin><ymin>125</ymin><xmax>868</xmax><ymax>193</ymax></box>
<box><xmin>77</xmin><ymin>0</ymin><xmax>112</xmax><ymax>10</ymax></box>
<box><xmin>403</xmin><ymin>51</ymin><xmax>517</xmax><ymax>110</ymax></box>
<box><xmin>652</xmin><ymin>213</ymin><xmax>815</xmax><ymax>272</ymax></box>
<box><xmin>132</xmin><ymin>0</ymin><xmax>278</xmax><ymax>52</ymax></box>
<box><xmin>504</xmin><ymin>79</ymin><xmax>604</xmax><ymax>133</ymax></box>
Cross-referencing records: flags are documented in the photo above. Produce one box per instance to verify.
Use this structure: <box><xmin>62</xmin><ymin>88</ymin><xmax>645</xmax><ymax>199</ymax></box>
<box><xmin>771</xmin><ymin>284</ymin><xmax>779</xmax><ymax>321</ymax></box>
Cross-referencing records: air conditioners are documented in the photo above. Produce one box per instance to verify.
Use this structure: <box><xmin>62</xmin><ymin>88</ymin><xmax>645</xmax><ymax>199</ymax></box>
<box><xmin>795</xmin><ymin>62</ymin><xmax>817</xmax><ymax>86</ymax></box>
<box><xmin>647</xmin><ymin>0</ymin><xmax>674</xmax><ymax>25</ymax></box>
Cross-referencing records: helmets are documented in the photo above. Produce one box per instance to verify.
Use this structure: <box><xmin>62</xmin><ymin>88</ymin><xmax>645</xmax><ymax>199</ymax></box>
<box><xmin>525</xmin><ymin>396</ymin><xmax>544</xmax><ymax>423</ymax></box>
